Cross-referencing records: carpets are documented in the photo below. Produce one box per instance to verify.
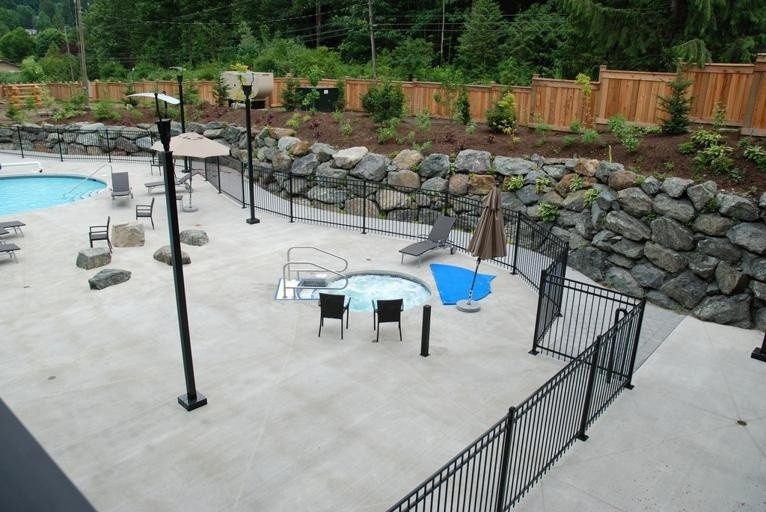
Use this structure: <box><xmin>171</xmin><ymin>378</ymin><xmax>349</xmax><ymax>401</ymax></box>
<box><xmin>430</xmin><ymin>264</ymin><xmax>496</xmax><ymax>305</ymax></box>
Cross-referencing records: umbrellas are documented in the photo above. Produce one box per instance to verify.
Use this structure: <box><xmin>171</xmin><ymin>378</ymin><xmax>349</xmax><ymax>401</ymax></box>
<box><xmin>467</xmin><ymin>182</ymin><xmax>507</xmax><ymax>304</ymax></box>
<box><xmin>149</xmin><ymin>130</ymin><xmax>231</xmax><ymax>206</ymax></box>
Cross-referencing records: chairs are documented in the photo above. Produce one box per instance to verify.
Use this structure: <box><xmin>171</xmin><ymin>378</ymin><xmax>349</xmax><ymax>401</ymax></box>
<box><xmin>318</xmin><ymin>293</ymin><xmax>350</xmax><ymax>340</ymax></box>
<box><xmin>136</xmin><ymin>197</ymin><xmax>154</xmax><ymax>229</ymax></box>
<box><xmin>0</xmin><ymin>221</ymin><xmax>26</xmax><ymax>264</ymax></box>
<box><xmin>144</xmin><ymin>170</ymin><xmax>200</xmax><ymax>194</ymax></box>
<box><xmin>89</xmin><ymin>216</ymin><xmax>113</xmax><ymax>254</ymax></box>
<box><xmin>371</xmin><ymin>299</ymin><xmax>403</xmax><ymax>342</ymax></box>
<box><xmin>398</xmin><ymin>216</ymin><xmax>458</xmax><ymax>267</ymax></box>
<box><xmin>110</xmin><ymin>172</ymin><xmax>133</xmax><ymax>200</ymax></box>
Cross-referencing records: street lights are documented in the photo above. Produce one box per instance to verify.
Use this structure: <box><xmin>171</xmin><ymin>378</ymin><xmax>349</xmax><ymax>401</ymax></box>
<box><xmin>239</xmin><ymin>72</ymin><xmax>262</xmax><ymax>225</ymax></box>
<box><xmin>125</xmin><ymin>89</ymin><xmax>209</xmax><ymax>414</ymax></box>
<box><xmin>168</xmin><ymin>65</ymin><xmax>192</xmax><ymax>173</ymax></box>
